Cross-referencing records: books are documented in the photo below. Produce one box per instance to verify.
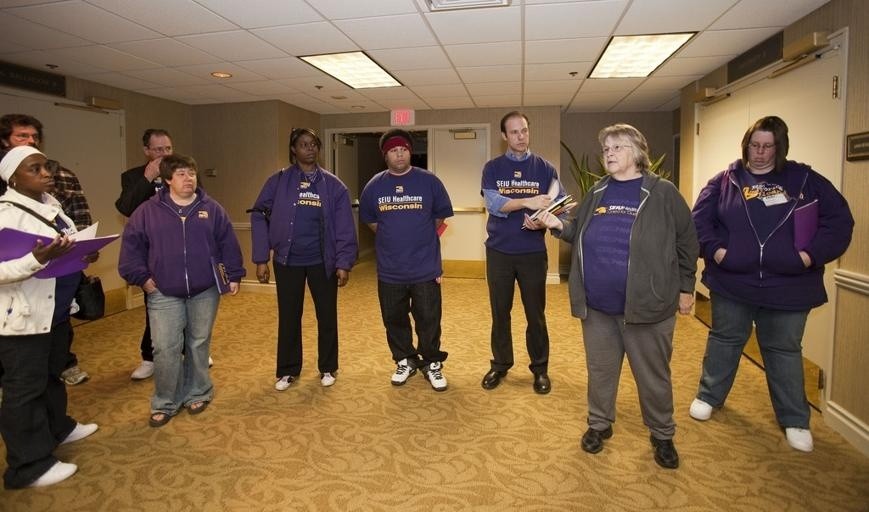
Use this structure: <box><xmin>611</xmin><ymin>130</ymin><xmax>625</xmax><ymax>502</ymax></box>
<box><xmin>522</xmin><ymin>194</ymin><xmax>579</xmax><ymax>230</ymax></box>
<box><xmin>0</xmin><ymin>220</ymin><xmax>120</xmax><ymax>280</ymax></box>
<box><xmin>210</xmin><ymin>257</ymin><xmax>231</xmax><ymax>295</ymax></box>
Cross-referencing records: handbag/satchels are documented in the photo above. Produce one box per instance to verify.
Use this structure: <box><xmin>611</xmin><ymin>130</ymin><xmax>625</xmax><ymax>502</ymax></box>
<box><xmin>74</xmin><ymin>272</ymin><xmax>105</xmax><ymax>324</ymax></box>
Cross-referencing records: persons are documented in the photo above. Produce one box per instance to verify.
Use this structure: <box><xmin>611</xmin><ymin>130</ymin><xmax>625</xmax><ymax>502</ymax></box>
<box><xmin>118</xmin><ymin>152</ymin><xmax>246</xmax><ymax>427</ymax></box>
<box><xmin>246</xmin><ymin>126</ymin><xmax>359</xmax><ymax>392</ymax></box>
<box><xmin>1</xmin><ymin>114</ymin><xmax>93</xmax><ymax>387</ymax></box>
<box><xmin>115</xmin><ymin>127</ymin><xmax>214</xmax><ymax>382</ymax></box>
<box><xmin>0</xmin><ymin>145</ymin><xmax>100</xmax><ymax>490</ymax></box>
<box><xmin>689</xmin><ymin>115</ymin><xmax>855</xmax><ymax>453</ymax></box>
<box><xmin>480</xmin><ymin>110</ymin><xmax>570</xmax><ymax>395</ymax></box>
<box><xmin>359</xmin><ymin>128</ymin><xmax>455</xmax><ymax>392</ymax></box>
<box><xmin>537</xmin><ymin>122</ymin><xmax>699</xmax><ymax>470</ymax></box>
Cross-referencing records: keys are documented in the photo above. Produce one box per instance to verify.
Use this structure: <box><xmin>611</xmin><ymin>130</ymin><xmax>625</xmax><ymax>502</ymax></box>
<box><xmin>435</xmin><ymin>273</ymin><xmax>444</xmax><ymax>284</ymax></box>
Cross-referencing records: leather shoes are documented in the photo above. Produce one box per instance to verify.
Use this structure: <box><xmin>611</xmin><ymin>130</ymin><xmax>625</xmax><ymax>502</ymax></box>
<box><xmin>581</xmin><ymin>424</ymin><xmax>613</xmax><ymax>454</ymax></box>
<box><xmin>481</xmin><ymin>367</ymin><xmax>508</xmax><ymax>390</ymax></box>
<box><xmin>649</xmin><ymin>432</ymin><xmax>679</xmax><ymax>469</ymax></box>
<box><xmin>532</xmin><ymin>368</ymin><xmax>551</xmax><ymax>394</ymax></box>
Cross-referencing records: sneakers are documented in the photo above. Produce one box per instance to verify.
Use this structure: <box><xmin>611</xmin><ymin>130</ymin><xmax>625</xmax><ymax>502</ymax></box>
<box><xmin>60</xmin><ymin>365</ymin><xmax>89</xmax><ymax>386</ymax></box>
<box><xmin>59</xmin><ymin>421</ymin><xmax>99</xmax><ymax>446</ymax></box>
<box><xmin>274</xmin><ymin>374</ymin><xmax>297</xmax><ymax>391</ymax></box>
<box><xmin>421</xmin><ymin>361</ymin><xmax>448</xmax><ymax>392</ymax></box>
<box><xmin>31</xmin><ymin>458</ymin><xmax>78</xmax><ymax>489</ymax></box>
<box><xmin>390</xmin><ymin>358</ymin><xmax>417</xmax><ymax>386</ymax></box>
<box><xmin>131</xmin><ymin>360</ymin><xmax>155</xmax><ymax>379</ymax></box>
<box><xmin>784</xmin><ymin>426</ymin><xmax>814</xmax><ymax>453</ymax></box>
<box><xmin>688</xmin><ymin>397</ymin><xmax>713</xmax><ymax>420</ymax></box>
<box><xmin>320</xmin><ymin>370</ymin><xmax>336</xmax><ymax>387</ymax></box>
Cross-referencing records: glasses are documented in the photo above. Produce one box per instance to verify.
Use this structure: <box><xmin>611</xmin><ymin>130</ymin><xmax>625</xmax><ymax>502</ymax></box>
<box><xmin>602</xmin><ymin>144</ymin><xmax>632</xmax><ymax>153</ymax></box>
<box><xmin>747</xmin><ymin>143</ymin><xmax>772</xmax><ymax>148</ymax></box>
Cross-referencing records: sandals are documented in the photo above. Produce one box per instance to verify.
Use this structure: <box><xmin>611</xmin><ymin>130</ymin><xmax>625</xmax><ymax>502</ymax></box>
<box><xmin>188</xmin><ymin>401</ymin><xmax>208</xmax><ymax>414</ymax></box>
<box><xmin>150</xmin><ymin>409</ymin><xmax>170</xmax><ymax>426</ymax></box>
